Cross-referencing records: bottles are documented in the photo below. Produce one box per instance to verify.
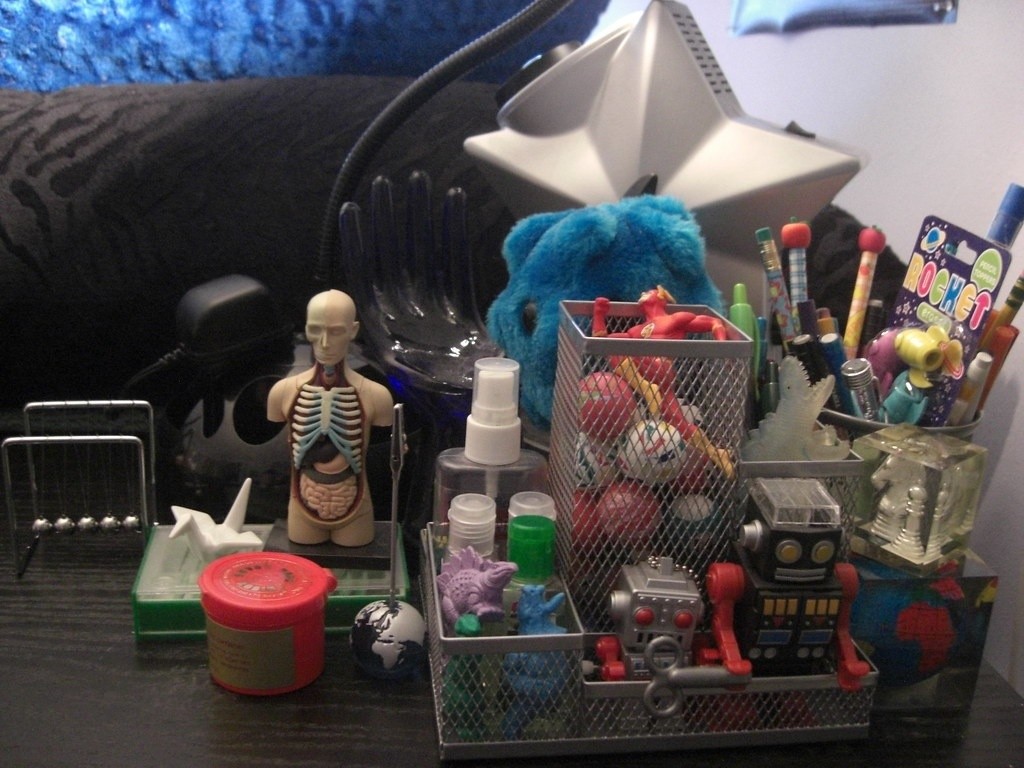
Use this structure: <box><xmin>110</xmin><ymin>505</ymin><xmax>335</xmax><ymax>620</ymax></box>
<box><xmin>433</xmin><ymin>362</ymin><xmax>561</xmax><ymax>637</ymax></box>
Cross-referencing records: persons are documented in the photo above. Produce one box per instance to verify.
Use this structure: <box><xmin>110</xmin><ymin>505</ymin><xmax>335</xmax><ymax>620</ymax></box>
<box><xmin>591</xmin><ymin>284</ymin><xmax>733</xmax><ymax>476</ymax></box>
<box><xmin>267</xmin><ymin>290</ymin><xmax>395</xmax><ymax>546</ymax></box>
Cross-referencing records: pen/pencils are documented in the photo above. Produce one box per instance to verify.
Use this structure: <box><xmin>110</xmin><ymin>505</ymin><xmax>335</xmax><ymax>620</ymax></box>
<box><xmin>726</xmin><ymin>281</ymin><xmax>780</xmax><ymax>430</ymax></box>
<box><xmin>755</xmin><ymin>179</ymin><xmax>1024</xmax><ymax>428</ymax></box>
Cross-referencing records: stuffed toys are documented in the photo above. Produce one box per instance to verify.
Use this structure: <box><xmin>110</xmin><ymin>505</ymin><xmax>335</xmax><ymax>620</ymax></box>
<box><xmin>486</xmin><ymin>196</ymin><xmax>722</xmax><ymax>432</ymax></box>
